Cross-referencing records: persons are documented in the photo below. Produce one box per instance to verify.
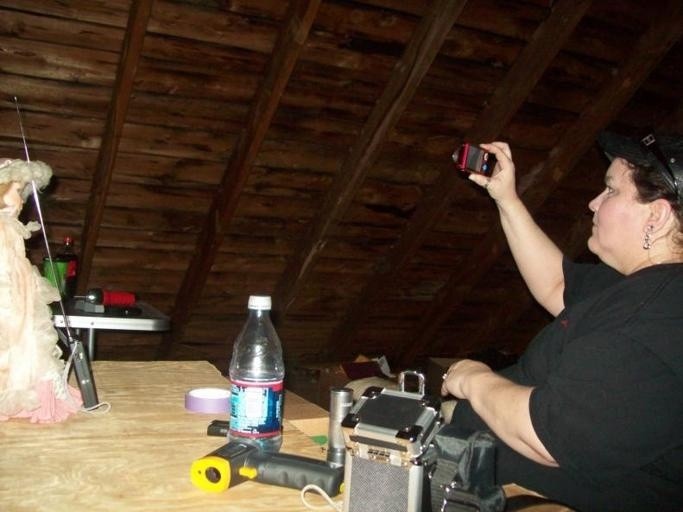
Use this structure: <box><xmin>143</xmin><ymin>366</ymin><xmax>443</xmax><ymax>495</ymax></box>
<box><xmin>0</xmin><ymin>156</ymin><xmax>82</xmax><ymax>425</ymax></box>
<box><xmin>439</xmin><ymin>125</ymin><xmax>683</xmax><ymax>511</ymax></box>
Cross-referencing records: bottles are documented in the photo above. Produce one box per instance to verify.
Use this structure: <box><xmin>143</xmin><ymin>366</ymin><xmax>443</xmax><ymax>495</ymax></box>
<box><xmin>56</xmin><ymin>235</ymin><xmax>78</xmax><ymax>296</ymax></box>
<box><xmin>227</xmin><ymin>294</ymin><xmax>288</xmax><ymax>452</ymax></box>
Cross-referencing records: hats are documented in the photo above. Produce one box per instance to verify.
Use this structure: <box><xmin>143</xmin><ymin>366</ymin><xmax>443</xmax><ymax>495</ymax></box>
<box><xmin>597</xmin><ymin>130</ymin><xmax>682</xmax><ymax>196</ymax></box>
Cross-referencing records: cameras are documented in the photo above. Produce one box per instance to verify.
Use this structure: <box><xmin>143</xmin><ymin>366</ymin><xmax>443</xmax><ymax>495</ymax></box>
<box><xmin>450</xmin><ymin>143</ymin><xmax>492</xmax><ymax>176</ymax></box>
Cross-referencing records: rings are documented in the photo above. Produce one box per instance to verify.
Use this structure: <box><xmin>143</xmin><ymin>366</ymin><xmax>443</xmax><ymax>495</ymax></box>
<box><xmin>440</xmin><ymin>369</ymin><xmax>447</xmax><ymax>380</ymax></box>
